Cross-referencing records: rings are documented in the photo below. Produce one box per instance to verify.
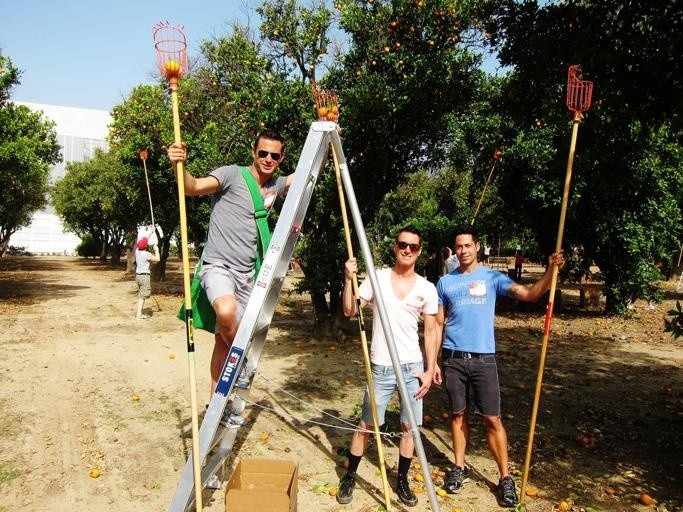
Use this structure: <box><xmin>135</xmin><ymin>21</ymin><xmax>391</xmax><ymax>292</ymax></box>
<box><xmin>560</xmin><ymin>257</ymin><xmax>564</xmax><ymax>261</ymax></box>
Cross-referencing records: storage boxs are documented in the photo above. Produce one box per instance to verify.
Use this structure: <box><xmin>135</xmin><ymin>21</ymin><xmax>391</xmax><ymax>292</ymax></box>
<box><xmin>224</xmin><ymin>459</ymin><xmax>297</xmax><ymax>512</ymax></box>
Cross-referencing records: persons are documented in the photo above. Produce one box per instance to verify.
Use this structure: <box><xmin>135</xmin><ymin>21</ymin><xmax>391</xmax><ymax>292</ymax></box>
<box><xmin>132</xmin><ymin>227</ymin><xmax>161</xmax><ymax>320</ymax></box>
<box><xmin>336</xmin><ymin>223</ymin><xmax>439</xmax><ymax>508</ymax></box>
<box><xmin>483</xmin><ymin>244</ymin><xmax>492</xmax><ymax>265</ymax></box>
<box><xmin>432</xmin><ymin>225</ymin><xmax>566</xmax><ymax>508</ymax></box>
<box><xmin>423</xmin><ymin>246</ymin><xmax>439</xmax><ymax>287</ymax></box>
<box><xmin>165</xmin><ymin>125</ymin><xmax>344</xmax><ymax>429</ymax></box>
<box><xmin>442</xmin><ymin>246</ymin><xmax>460</xmax><ymax>276</ymax></box>
<box><xmin>513</xmin><ymin>250</ymin><xmax>526</xmax><ymax>280</ymax></box>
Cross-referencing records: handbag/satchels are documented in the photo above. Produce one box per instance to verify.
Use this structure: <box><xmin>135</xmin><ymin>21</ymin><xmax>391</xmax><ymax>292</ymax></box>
<box><xmin>175</xmin><ymin>257</ymin><xmax>218</xmax><ymax>337</ymax></box>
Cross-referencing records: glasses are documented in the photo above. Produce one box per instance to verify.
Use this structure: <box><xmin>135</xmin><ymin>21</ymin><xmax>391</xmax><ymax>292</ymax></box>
<box><xmin>396</xmin><ymin>241</ymin><xmax>420</xmax><ymax>253</ymax></box>
<box><xmin>256</xmin><ymin>149</ymin><xmax>281</xmax><ymax>162</ymax></box>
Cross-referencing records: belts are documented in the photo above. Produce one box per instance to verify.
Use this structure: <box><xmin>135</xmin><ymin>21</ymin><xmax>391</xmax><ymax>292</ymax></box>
<box><xmin>135</xmin><ymin>273</ymin><xmax>150</xmax><ymax>276</ymax></box>
<box><xmin>441</xmin><ymin>349</ymin><xmax>480</xmax><ymax>360</ymax></box>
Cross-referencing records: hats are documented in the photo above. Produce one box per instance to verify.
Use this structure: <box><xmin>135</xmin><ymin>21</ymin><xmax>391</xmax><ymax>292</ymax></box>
<box><xmin>135</xmin><ymin>237</ymin><xmax>148</xmax><ymax>250</ymax></box>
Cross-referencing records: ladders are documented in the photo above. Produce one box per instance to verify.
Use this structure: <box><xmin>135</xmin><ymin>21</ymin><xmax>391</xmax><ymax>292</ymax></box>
<box><xmin>168</xmin><ymin>122</ymin><xmax>442</xmax><ymax>512</ymax></box>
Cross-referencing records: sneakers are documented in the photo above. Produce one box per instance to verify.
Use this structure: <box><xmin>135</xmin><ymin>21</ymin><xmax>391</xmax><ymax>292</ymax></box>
<box><xmin>204</xmin><ymin>404</ymin><xmax>248</xmax><ymax>427</ymax></box>
<box><xmin>136</xmin><ymin>313</ymin><xmax>150</xmax><ymax>321</ymax></box>
<box><xmin>496</xmin><ymin>474</ymin><xmax>518</xmax><ymax>507</ymax></box>
<box><xmin>335</xmin><ymin>469</ymin><xmax>356</xmax><ymax>504</ymax></box>
<box><xmin>396</xmin><ymin>470</ymin><xmax>418</xmax><ymax>506</ymax></box>
<box><xmin>445</xmin><ymin>463</ymin><xmax>470</xmax><ymax>494</ymax></box>
<box><xmin>233</xmin><ymin>354</ymin><xmax>250</xmax><ymax>389</ymax></box>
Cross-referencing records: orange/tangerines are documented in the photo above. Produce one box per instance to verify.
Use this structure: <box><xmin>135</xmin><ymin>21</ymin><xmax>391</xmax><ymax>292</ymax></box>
<box><xmin>92</xmin><ymin>339</ymin><xmax>654</xmax><ymax>512</ymax></box>
<box><xmin>103</xmin><ymin>0</ymin><xmax>502</xmax><ymax>159</ymax></box>
